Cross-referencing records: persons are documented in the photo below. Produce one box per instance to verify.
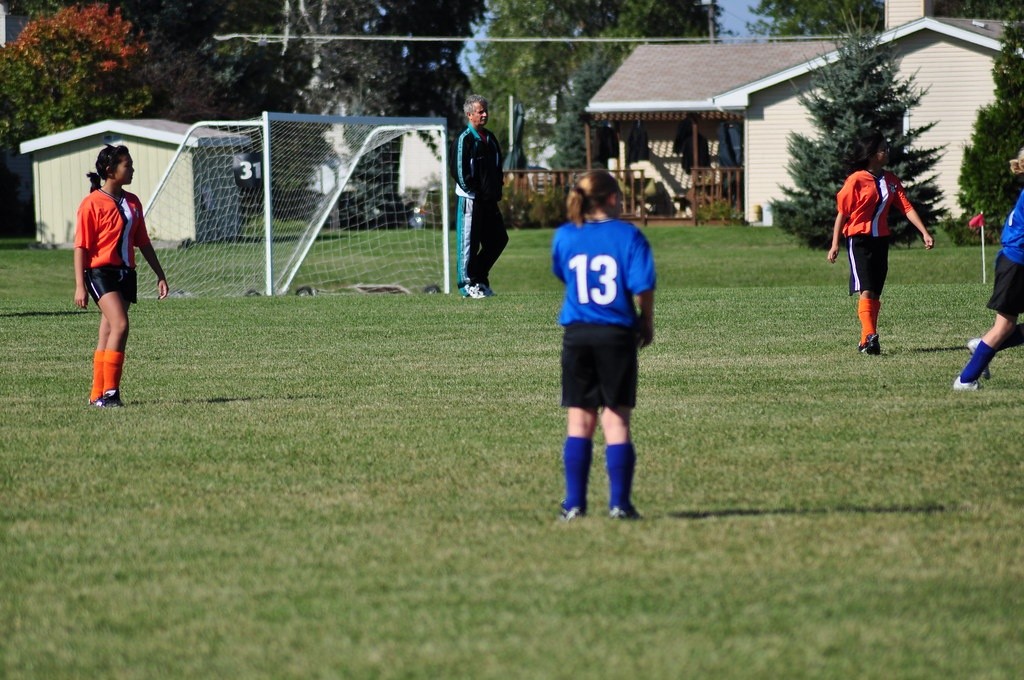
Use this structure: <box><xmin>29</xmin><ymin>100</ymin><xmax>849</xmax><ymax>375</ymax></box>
<box><xmin>952</xmin><ymin>146</ymin><xmax>1024</xmax><ymax>393</ymax></box>
<box><xmin>547</xmin><ymin>169</ymin><xmax>657</xmax><ymax>522</ymax></box>
<box><xmin>75</xmin><ymin>142</ymin><xmax>169</xmax><ymax>409</ymax></box>
<box><xmin>455</xmin><ymin>94</ymin><xmax>510</xmax><ymax>301</ymax></box>
<box><xmin>823</xmin><ymin>135</ymin><xmax>938</xmax><ymax>358</ymax></box>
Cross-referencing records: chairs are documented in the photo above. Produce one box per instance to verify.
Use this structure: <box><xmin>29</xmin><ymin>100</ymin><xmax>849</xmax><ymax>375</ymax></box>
<box><xmin>674</xmin><ymin>185</ymin><xmax>720</xmax><ymax>211</ymax></box>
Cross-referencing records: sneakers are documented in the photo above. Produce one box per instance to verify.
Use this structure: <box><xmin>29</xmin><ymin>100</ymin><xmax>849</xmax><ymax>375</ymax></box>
<box><xmin>952</xmin><ymin>376</ymin><xmax>980</xmax><ymax>391</ymax></box>
<box><xmin>88</xmin><ymin>388</ymin><xmax>124</xmax><ymax>408</ymax></box>
<box><xmin>858</xmin><ymin>333</ymin><xmax>881</xmax><ymax>355</ymax></box>
<box><xmin>967</xmin><ymin>337</ymin><xmax>992</xmax><ymax>379</ymax></box>
<box><xmin>559</xmin><ymin>497</ymin><xmax>585</xmax><ymax>523</ymax></box>
<box><xmin>468</xmin><ymin>283</ymin><xmax>496</xmax><ymax>298</ymax></box>
<box><xmin>609</xmin><ymin>506</ymin><xmax>641</xmax><ymax>520</ymax></box>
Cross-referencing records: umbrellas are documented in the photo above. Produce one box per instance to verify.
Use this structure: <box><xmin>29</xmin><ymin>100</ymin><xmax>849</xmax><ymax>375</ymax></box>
<box><xmin>501</xmin><ymin>99</ymin><xmax>529</xmax><ymax>197</ymax></box>
